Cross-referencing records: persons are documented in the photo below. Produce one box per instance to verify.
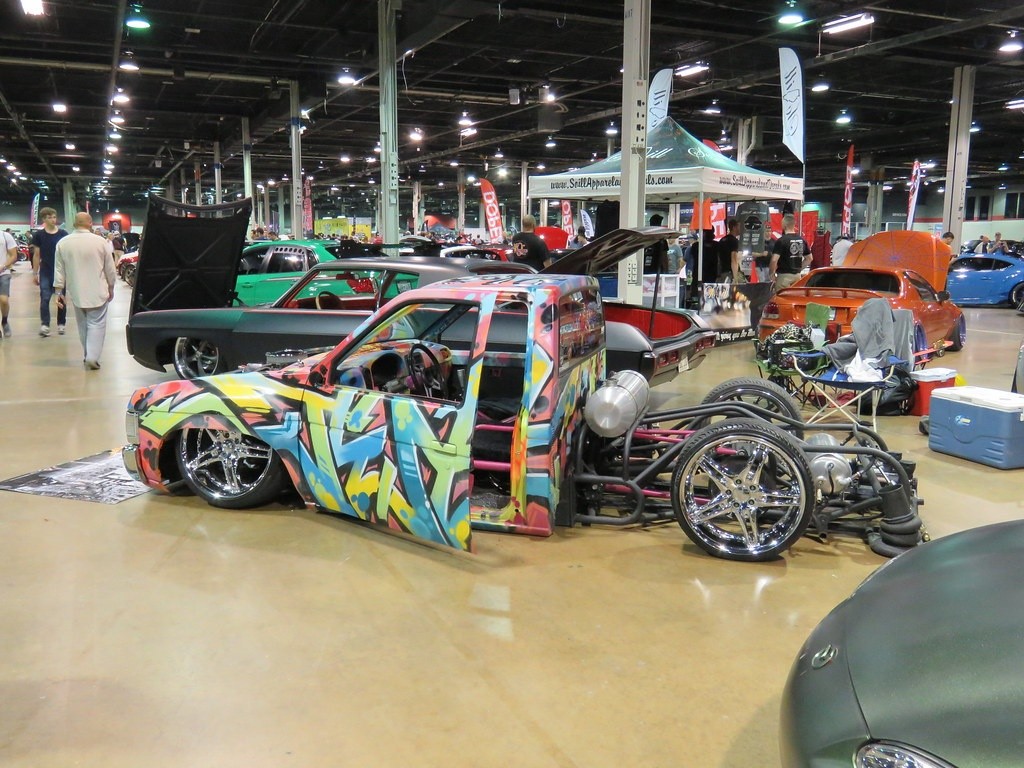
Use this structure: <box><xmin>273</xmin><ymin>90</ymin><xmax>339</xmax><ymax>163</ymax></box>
<box><xmin>0</xmin><ymin>231</ymin><xmax>17</xmax><ymax>339</ymax></box>
<box><xmin>249</xmin><ymin>224</ymin><xmax>588</xmax><ymax>249</ymax></box>
<box><xmin>53</xmin><ymin>211</ymin><xmax>116</xmax><ymax>369</ymax></box>
<box><xmin>941</xmin><ymin>232</ymin><xmax>959</xmax><ymax>260</ymax></box>
<box><xmin>974</xmin><ymin>235</ymin><xmax>990</xmax><ymax>256</ymax></box>
<box><xmin>512</xmin><ymin>214</ymin><xmax>551</xmax><ymax>270</ymax></box>
<box><xmin>643</xmin><ymin>214</ymin><xmax>858</xmax><ymax>306</ymax></box>
<box><xmin>6</xmin><ymin>228</ymin><xmax>142</xmax><ymax>270</ymax></box>
<box><xmin>988</xmin><ymin>232</ymin><xmax>1009</xmax><ymax>256</ymax></box>
<box><xmin>32</xmin><ymin>207</ymin><xmax>69</xmax><ymax>337</ymax></box>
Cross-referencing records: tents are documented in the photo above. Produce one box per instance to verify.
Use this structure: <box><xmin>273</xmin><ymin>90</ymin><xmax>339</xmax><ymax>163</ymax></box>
<box><xmin>528</xmin><ymin>118</ymin><xmax>803</xmax><ymax>316</ymax></box>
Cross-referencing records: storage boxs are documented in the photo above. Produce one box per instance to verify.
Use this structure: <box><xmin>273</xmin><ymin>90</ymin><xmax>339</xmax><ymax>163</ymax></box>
<box><xmin>909</xmin><ymin>368</ymin><xmax>957</xmax><ymax>417</ymax></box>
<box><xmin>929</xmin><ymin>386</ymin><xmax>1024</xmax><ymax>468</ymax></box>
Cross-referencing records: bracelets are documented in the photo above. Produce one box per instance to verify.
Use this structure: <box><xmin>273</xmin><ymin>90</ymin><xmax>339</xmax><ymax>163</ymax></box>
<box><xmin>55</xmin><ymin>291</ymin><xmax>62</xmax><ymax>295</ymax></box>
<box><xmin>3</xmin><ymin>265</ymin><xmax>6</xmax><ymax>272</ymax></box>
<box><xmin>33</xmin><ymin>272</ymin><xmax>38</xmax><ymax>275</ymax></box>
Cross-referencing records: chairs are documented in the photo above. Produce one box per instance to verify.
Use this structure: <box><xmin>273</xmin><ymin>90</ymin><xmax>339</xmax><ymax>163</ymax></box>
<box><xmin>751</xmin><ymin>298</ymin><xmax>954</xmax><ymax>435</ymax></box>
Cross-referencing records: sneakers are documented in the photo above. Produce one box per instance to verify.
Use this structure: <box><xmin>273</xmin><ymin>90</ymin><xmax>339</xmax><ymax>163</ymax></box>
<box><xmin>86</xmin><ymin>360</ymin><xmax>101</xmax><ymax>369</ymax></box>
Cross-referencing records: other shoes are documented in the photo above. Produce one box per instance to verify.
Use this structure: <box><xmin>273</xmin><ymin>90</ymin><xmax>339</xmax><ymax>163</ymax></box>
<box><xmin>39</xmin><ymin>325</ymin><xmax>51</xmax><ymax>337</ymax></box>
<box><xmin>1</xmin><ymin>322</ymin><xmax>11</xmax><ymax>336</ymax></box>
<box><xmin>57</xmin><ymin>324</ymin><xmax>64</xmax><ymax>333</ymax></box>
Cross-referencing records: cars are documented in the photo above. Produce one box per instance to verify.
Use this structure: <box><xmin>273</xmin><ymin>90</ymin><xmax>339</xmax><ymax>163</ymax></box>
<box><xmin>778</xmin><ymin>519</ymin><xmax>1023</xmax><ymax>768</ymax></box>
<box><xmin>116</xmin><ymin>251</ymin><xmax>139</xmax><ymax>287</ymax></box>
<box><xmin>126</xmin><ymin>188</ymin><xmax>717</xmax><ymax>389</ymax></box>
<box><xmin>231</xmin><ymin>237</ymin><xmax>419</xmax><ymax>306</ymax></box>
<box><xmin>961</xmin><ymin>240</ymin><xmax>1024</xmax><ymax>256</ymax></box>
<box><xmin>15</xmin><ymin>238</ymin><xmax>30</xmax><ymax>261</ymax></box>
<box><xmin>756</xmin><ymin>231</ymin><xmax>967</xmax><ymax>372</ymax></box>
<box><xmin>945</xmin><ymin>253</ymin><xmax>1024</xmax><ymax>310</ymax></box>
<box><xmin>398</xmin><ymin>227</ymin><xmax>576</xmax><ymax>263</ymax></box>
